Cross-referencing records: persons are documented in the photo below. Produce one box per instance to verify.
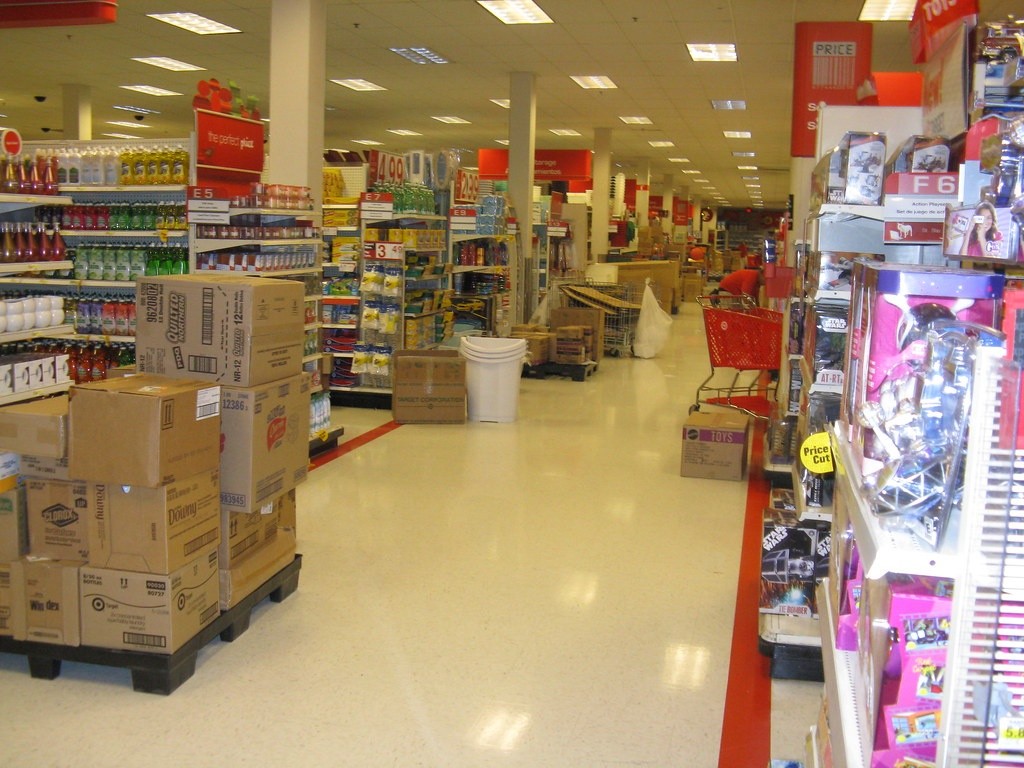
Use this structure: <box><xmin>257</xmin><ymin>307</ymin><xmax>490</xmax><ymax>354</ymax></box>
<box><xmin>958</xmin><ymin>203</ymin><xmax>1004</xmax><ymax>258</ymax></box>
<box><xmin>716</xmin><ymin>269</ymin><xmax>767</xmax><ymax>313</ymax></box>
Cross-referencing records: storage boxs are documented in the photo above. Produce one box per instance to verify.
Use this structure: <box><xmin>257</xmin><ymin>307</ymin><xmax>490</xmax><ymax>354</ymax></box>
<box><xmin>681</xmin><ymin>249</ymin><xmax>743</xmax><ymax>303</ymax></box>
<box><xmin>511</xmin><ymin>306</ymin><xmax>606</xmax><ymax>365</ymax></box>
<box><xmin>0</xmin><ymin>274</ymin><xmax>314</xmax><ymax>660</ymax></box>
<box><xmin>761</xmin><ymin>20</ymin><xmax>1024</xmax><ymax>768</ymax></box>
<box><xmin>681</xmin><ymin>411</ymin><xmax>750</xmax><ymax>482</ymax></box>
<box><xmin>196</xmin><ymin>182</ymin><xmax>319</xmax><ymax>210</ymax></box>
<box><xmin>197</xmin><ymin>219</ymin><xmax>321</xmax><ymax>240</ymax></box>
<box><xmin>637</xmin><ymin>226</ymin><xmax>664</xmax><ymax>255</ymax></box>
<box><xmin>323</xmin><ymin>195</ymin><xmax>468</xmax><ymax>425</ymax></box>
<box><xmin>196</xmin><ymin>245</ymin><xmax>317</xmax><ymax>270</ymax></box>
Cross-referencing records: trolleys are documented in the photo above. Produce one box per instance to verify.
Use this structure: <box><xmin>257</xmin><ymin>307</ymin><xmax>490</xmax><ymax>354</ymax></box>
<box><xmin>688</xmin><ymin>292</ymin><xmax>792</xmax><ymax>425</ymax></box>
<box><xmin>554</xmin><ymin>275</ymin><xmax>664</xmax><ymax>358</ymax></box>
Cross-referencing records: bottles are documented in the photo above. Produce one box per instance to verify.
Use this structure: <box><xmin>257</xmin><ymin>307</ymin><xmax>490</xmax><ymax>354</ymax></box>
<box><xmin>371</xmin><ymin>180</ymin><xmax>435</xmax><ymax>218</ymax></box>
<box><xmin>1</xmin><ymin>143</ymin><xmax>330</xmax><ymax>440</ymax></box>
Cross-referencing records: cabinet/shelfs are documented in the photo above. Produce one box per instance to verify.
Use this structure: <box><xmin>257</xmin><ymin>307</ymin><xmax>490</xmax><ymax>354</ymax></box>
<box><xmin>4</xmin><ymin>132</ymin><xmax>345</xmax><ymax>459</ymax></box>
<box><xmin>0</xmin><ymin>193</ymin><xmax>78</xmax><ymax>408</ymax></box>
<box><xmin>707</xmin><ymin>229</ymin><xmax>727</xmax><ymax>251</ymax></box>
<box><xmin>811</xmin><ymin>336</ymin><xmax>1024</xmax><ymax>768</ymax></box>
<box><xmin>453</xmin><ymin>216</ymin><xmax>524</xmax><ymax>338</ymax></box>
<box><xmin>560</xmin><ymin>202</ymin><xmax>593</xmax><ymax>269</ymax></box>
<box><xmin>530</xmin><ymin>200</ymin><xmax>567</xmax><ymax>323</ymax></box>
<box><xmin>729</xmin><ymin>230</ymin><xmax>764</xmax><ymax>250</ymax></box>
<box><xmin>754</xmin><ymin>160</ymin><xmax>981</xmax><ymax>703</ymax></box>
<box><xmin>323</xmin><ymin>160</ymin><xmax>456</xmax><ymax>412</ymax></box>
